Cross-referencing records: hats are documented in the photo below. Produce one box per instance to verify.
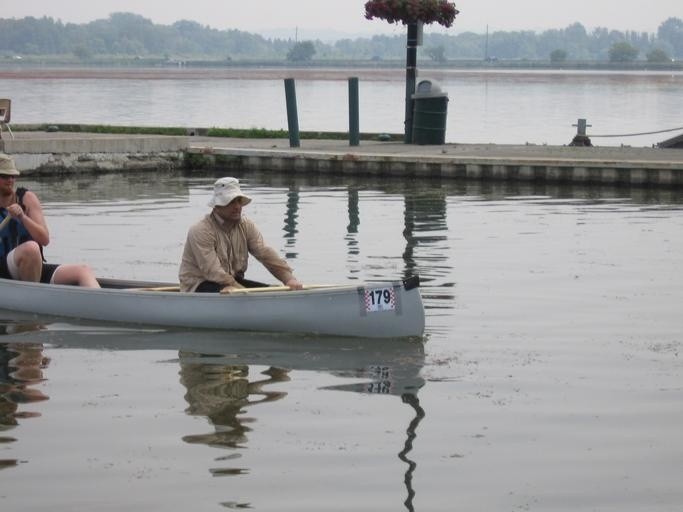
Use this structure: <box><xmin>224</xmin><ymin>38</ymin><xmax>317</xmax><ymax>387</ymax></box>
<box><xmin>0</xmin><ymin>154</ymin><xmax>21</xmax><ymax>177</ymax></box>
<box><xmin>205</xmin><ymin>176</ymin><xmax>252</xmax><ymax>209</ymax></box>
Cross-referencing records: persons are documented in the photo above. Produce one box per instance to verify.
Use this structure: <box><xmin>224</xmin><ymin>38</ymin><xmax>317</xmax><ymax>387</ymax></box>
<box><xmin>178</xmin><ymin>176</ymin><xmax>303</xmax><ymax>293</ymax></box>
<box><xmin>0</xmin><ymin>322</ymin><xmax>47</xmax><ymax>437</ymax></box>
<box><xmin>0</xmin><ymin>155</ymin><xmax>101</xmax><ymax>290</ymax></box>
<box><xmin>179</xmin><ymin>347</ymin><xmax>254</xmax><ymax>449</ymax></box>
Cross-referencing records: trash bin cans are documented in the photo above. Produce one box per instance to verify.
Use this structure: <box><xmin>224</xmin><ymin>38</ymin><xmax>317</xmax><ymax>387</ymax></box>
<box><xmin>411</xmin><ymin>79</ymin><xmax>448</xmax><ymax>144</ymax></box>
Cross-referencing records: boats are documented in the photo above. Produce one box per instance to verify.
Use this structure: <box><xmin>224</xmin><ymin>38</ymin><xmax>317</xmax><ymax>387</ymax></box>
<box><xmin>0</xmin><ymin>274</ymin><xmax>425</xmax><ymax>339</ymax></box>
